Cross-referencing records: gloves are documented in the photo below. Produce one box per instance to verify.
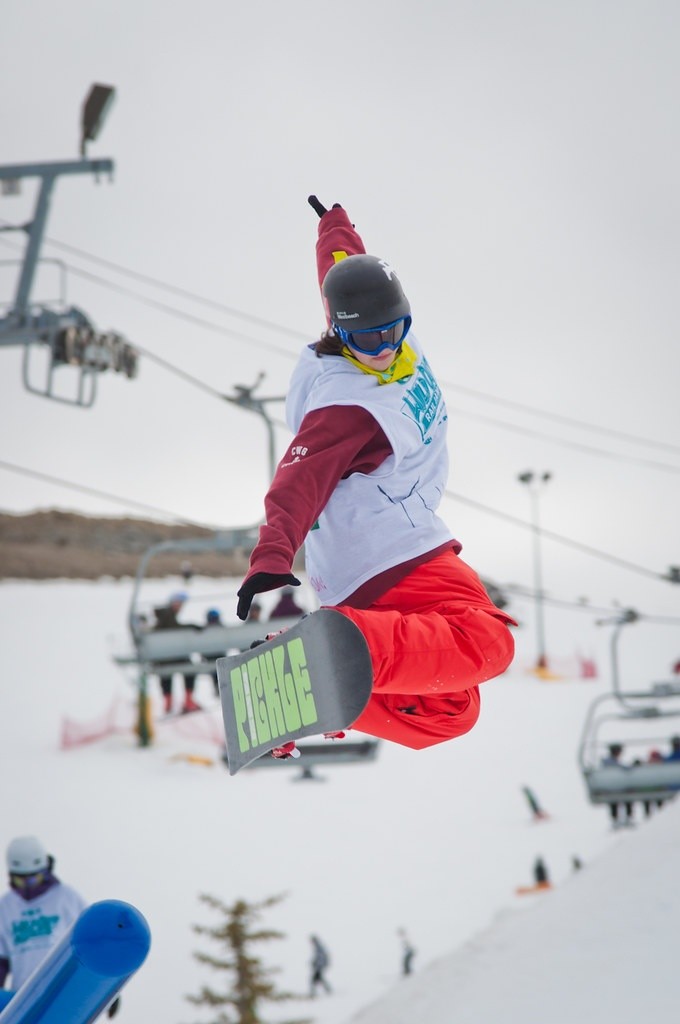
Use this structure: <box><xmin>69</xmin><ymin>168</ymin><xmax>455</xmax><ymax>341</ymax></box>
<box><xmin>234</xmin><ymin>570</ymin><xmax>302</xmax><ymax>620</ymax></box>
<box><xmin>308</xmin><ymin>195</ymin><xmax>353</xmax><ymax>227</ymax></box>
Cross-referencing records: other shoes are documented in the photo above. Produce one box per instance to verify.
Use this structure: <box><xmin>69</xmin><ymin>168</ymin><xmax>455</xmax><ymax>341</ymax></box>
<box><xmin>184</xmin><ymin>701</ymin><xmax>203</xmax><ymax>712</ymax></box>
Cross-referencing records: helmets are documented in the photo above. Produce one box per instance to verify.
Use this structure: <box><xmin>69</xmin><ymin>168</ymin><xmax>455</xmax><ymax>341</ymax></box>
<box><xmin>321</xmin><ymin>255</ymin><xmax>412</xmax><ymax>330</ymax></box>
<box><xmin>6</xmin><ymin>837</ymin><xmax>48</xmax><ymax>874</ymax></box>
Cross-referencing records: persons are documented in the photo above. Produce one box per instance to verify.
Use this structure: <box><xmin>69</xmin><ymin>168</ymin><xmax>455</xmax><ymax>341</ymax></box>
<box><xmin>245</xmin><ymin>603</ymin><xmax>262</xmax><ymax>624</ymax></box>
<box><xmin>151</xmin><ymin>592</ymin><xmax>201</xmax><ymax>713</ymax></box>
<box><xmin>401</xmin><ymin>944</ymin><xmax>413</xmax><ymax>973</ymax></box>
<box><xmin>535</xmin><ymin>859</ymin><xmax>546</xmax><ymax>884</ymax></box>
<box><xmin>237</xmin><ymin>197</ymin><xmax>515</xmax><ymax>757</ymax></box>
<box><xmin>307</xmin><ymin>936</ymin><xmax>330</xmax><ymax>997</ymax></box>
<box><xmin>603</xmin><ymin>736</ymin><xmax>680</xmax><ymax>823</ymax></box>
<box><xmin>267</xmin><ymin>586</ymin><xmax>301</xmax><ymax>617</ymax></box>
<box><xmin>0</xmin><ymin>836</ymin><xmax>84</xmax><ymax>994</ymax></box>
<box><xmin>525</xmin><ymin>787</ymin><xmax>539</xmax><ymax>813</ymax></box>
<box><xmin>202</xmin><ymin>610</ymin><xmax>229</xmax><ymax>694</ymax></box>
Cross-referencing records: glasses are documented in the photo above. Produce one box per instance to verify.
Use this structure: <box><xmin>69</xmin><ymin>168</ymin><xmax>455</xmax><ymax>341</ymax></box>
<box><xmin>346</xmin><ymin>315</ymin><xmax>414</xmax><ymax>355</ymax></box>
<box><xmin>13</xmin><ymin>871</ymin><xmax>44</xmax><ymax>888</ymax></box>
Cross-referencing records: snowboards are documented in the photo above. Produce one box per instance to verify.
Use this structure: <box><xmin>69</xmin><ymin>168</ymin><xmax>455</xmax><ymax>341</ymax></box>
<box><xmin>216</xmin><ymin>606</ymin><xmax>375</xmax><ymax>777</ymax></box>
